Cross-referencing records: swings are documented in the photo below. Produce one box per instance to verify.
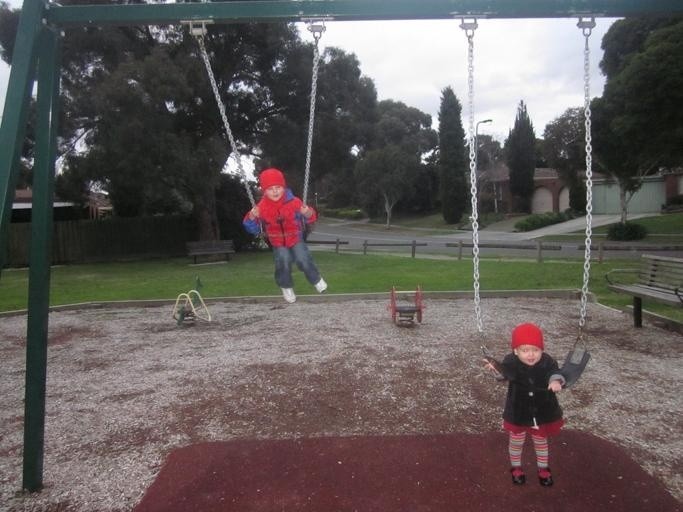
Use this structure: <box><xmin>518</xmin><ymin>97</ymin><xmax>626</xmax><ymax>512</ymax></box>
<box><xmin>453</xmin><ymin>12</ymin><xmax>604</xmax><ymax>388</ymax></box>
<box><xmin>181</xmin><ymin>18</ymin><xmax>336</xmax><ymax>250</ymax></box>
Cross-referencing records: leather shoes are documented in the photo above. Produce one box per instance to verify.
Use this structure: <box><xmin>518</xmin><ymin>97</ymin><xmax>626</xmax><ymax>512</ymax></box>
<box><xmin>536</xmin><ymin>466</ymin><xmax>553</xmax><ymax>487</ymax></box>
<box><xmin>510</xmin><ymin>465</ymin><xmax>526</xmax><ymax>485</ymax></box>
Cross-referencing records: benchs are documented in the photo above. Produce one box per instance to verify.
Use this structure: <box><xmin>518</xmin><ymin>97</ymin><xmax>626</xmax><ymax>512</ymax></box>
<box><xmin>603</xmin><ymin>254</ymin><xmax>683</xmax><ymax>329</ymax></box>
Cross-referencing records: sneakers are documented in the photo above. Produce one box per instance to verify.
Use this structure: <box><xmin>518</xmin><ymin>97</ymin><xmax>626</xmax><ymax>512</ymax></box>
<box><xmin>314</xmin><ymin>277</ymin><xmax>328</xmax><ymax>294</ymax></box>
<box><xmin>280</xmin><ymin>286</ymin><xmax>296</xmax><ymax>304</ymax></box>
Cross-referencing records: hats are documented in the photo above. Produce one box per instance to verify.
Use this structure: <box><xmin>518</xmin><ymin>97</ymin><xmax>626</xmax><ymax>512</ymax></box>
<box><xmin>259</xmin><ymin>167</ymin><xmax>286</xmax><ymax>191</ymax></box>
<box><xmin>511</xmin><ymin>323</ymin><xmax>544</xmax><ymax>350</ymax></box>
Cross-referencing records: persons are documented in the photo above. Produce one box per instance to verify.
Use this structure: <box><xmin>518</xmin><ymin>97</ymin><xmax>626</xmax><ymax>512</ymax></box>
<box><xmin>481</xmin><ymin>323</ymin><xmax>568</xmax><ymax>486</ymax></box>
<box><xmin>243</xmin><ymin>168</ymin><xmax>328</xmax><ymax>304</ymax></box>
